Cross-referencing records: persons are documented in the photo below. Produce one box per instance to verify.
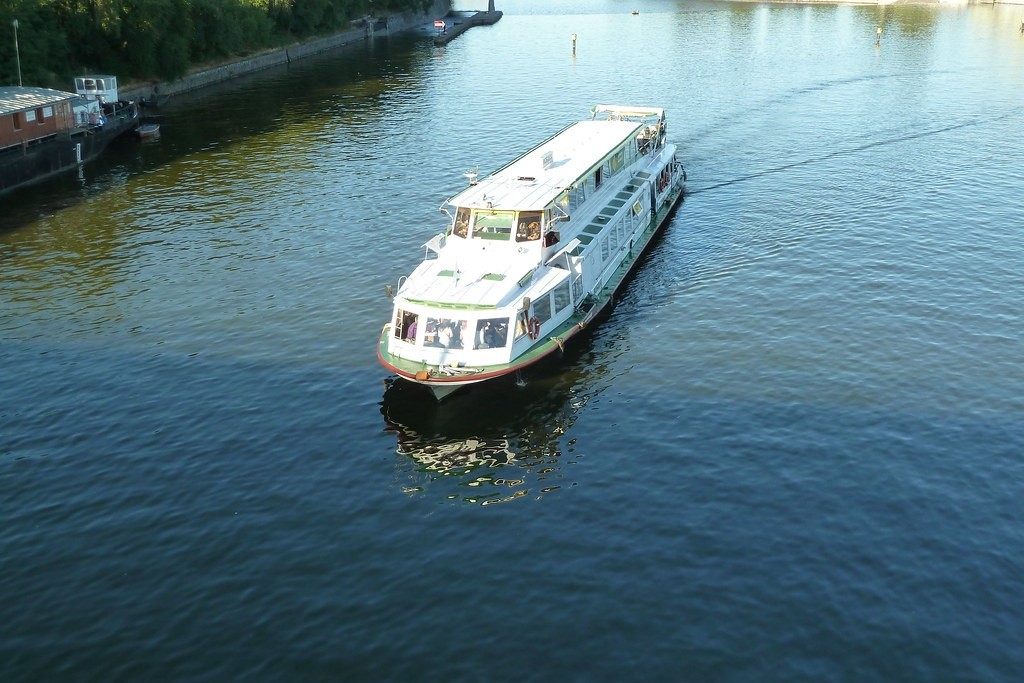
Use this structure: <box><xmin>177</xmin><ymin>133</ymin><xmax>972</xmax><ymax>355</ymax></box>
<box><xmin>438</xmin><ymin>319</ymin><xmax>453</xmax><ymax>348</ymax></box>
<box><xmin>460</xmin><ymin>314</ymin><xmax>508</xmax><ymax>348</ymax></box>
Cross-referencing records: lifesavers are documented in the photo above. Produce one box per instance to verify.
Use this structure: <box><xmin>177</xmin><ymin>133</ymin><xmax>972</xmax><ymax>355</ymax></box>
<box><xmin>666</xmin><ymin>175</ymin><xmax>669</xmax><ymax>183</ymax></box>
<box><xmin>660</xmin><ymin>178</ymin><xmax>664</xmax><ymax>192</ymax></box>
<box><xmin>528</xmin><ymin>316</ymin><xmax>540</xmax><ymax>339</ymax></box>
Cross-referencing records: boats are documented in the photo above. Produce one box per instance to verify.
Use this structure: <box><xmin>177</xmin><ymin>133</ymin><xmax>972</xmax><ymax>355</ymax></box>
<box><xmin>375</xmin><ymin>104</ymin><xmax>688</xmax><ymax>400</ymax></box>
